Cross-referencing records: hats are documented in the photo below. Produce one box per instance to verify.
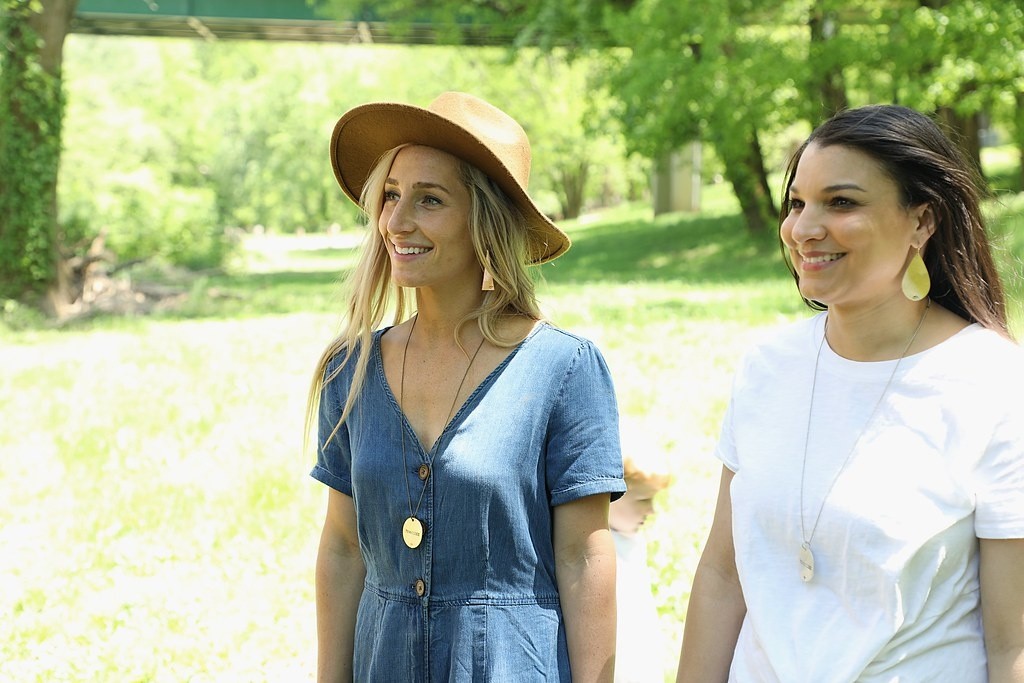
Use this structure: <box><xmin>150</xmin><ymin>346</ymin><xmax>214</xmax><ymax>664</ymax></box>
<box><xmin>329</xmin><ymin>91</ymin><xmax>571</xmax><ymax>267</ymax></box>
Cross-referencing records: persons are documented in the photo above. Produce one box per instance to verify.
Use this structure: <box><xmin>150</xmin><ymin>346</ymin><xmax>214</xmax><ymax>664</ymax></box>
<box><xmin>677</xmin><ymin>106</ymin><xmax>1024</xmax><ymax>683</ymax></box>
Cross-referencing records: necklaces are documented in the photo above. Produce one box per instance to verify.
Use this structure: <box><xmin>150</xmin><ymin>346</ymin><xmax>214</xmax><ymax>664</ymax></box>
<box><xmin>399</xmin><ymin>312</ymin><xmax>499</xmax><ymax>550</ymax></box>
<box><xmin>797</xmin><ymin>295</ymin><xmax>933</xmax><ymax>581</ymax></box>
<box><xmin>306</xmin><ymin>89</ymin><xmax>629</xmax><ymax>683</ymax></box>
